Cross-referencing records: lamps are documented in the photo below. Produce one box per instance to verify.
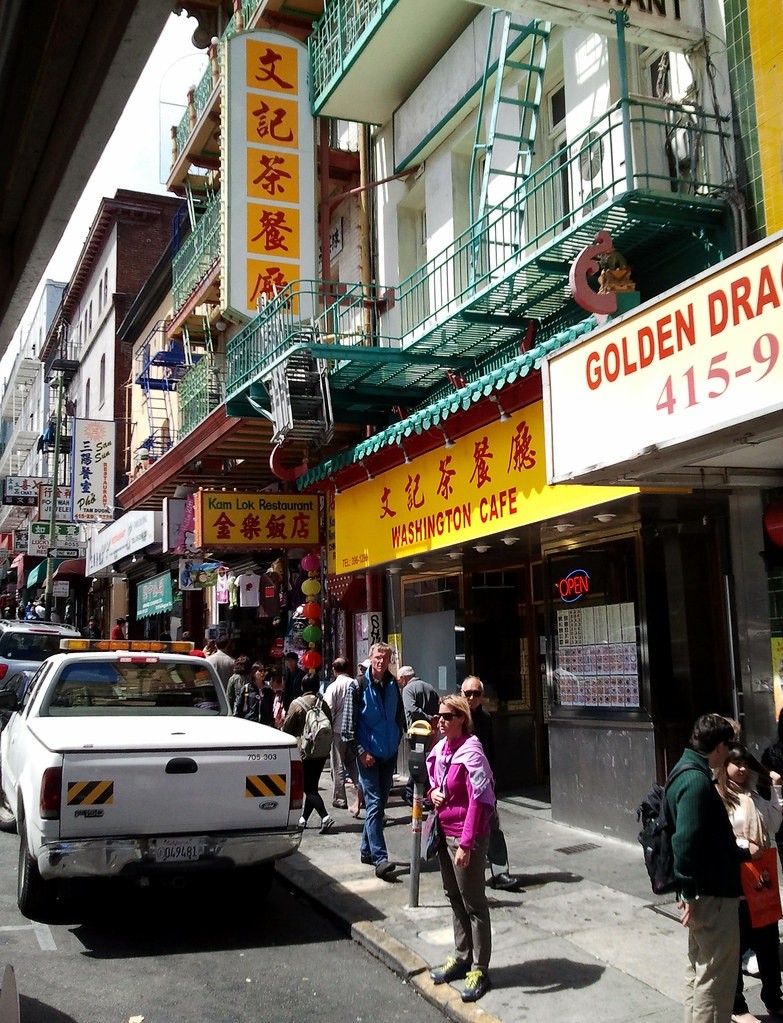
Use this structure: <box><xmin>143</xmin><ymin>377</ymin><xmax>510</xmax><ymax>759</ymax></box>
<box><xmin>386</xmin><ymin>568</ymin><xmax>402</xmax><ymax>573</ymax></box>
<box><xmin>554</xmin><ymin>524</ymin><xmax>574</xmax><ymax>531</ymax></box>
<box><xmin>409</xmin><ymin>562</ymin><xmax>424</xmax><ymax>569</ymax></box>
<box><xmin>216</xmin><ymin>314</ymin><xmax>226</xmax><ymax>330</ymax></box>
<box><xmin>488</xmin><ymin>396</ymin><xmax>513</xmax><ymax>422</ymax></box>
<box><xmin>398</xmin><ymin>443</ymin><xmax>413</xmax><ymax>463</ymax></box>
<box><xmin>359</xmin><ymin>461</ymin><xmax>375</xmax><ymax>480</ymax></box>
<box><xmin>93</xmin><ymin>574</ymin><xmax>97</xmax><ymax>582</ymax></box>
<box><xmin>132</xmin><ymin>554</ymin><xmax>136</xmax><ymax>562</ymax></box>
<box><xmin>445</xmin><ymin>552</ymin><xmax>464</xmax><ymax>560</ymax></box>
<box><xmin>501</xmin><ymin>538</ymin><xmax>520</xmax><ymax>545</ymax></box>
<box><xmin>110</xmin><ymin>565</ymin><xmax>116</xmax><ymax>573</ymax></box>
<box><xmin>436</xmin><ymin>423</ymin><xmax>457</xmax><ymax>449</ymax></box>
<box><xmin>593</xmin><ymin>514</ymin><xmax>616</xmax><ymax>522</ymax></box>
<box><xmin>330</xmin><ymin>476</ymin><xmax>342</xmax><ymax>495</ymax></box>
<box><xmin>473</xmin><ymin>546</ymin><xmax>492</xmax><ymax>553</ymax></box>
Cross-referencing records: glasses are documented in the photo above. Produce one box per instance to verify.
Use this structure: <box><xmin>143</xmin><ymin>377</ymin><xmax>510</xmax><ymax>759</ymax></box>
<box><xmin>436</xmin><ymin>712</ymin><xmax>462</xmax><ymax>722</ymax></box>
<box><xmin>462</xmin><ymin>689</ymin><xmax>482</xmax><ymax>697</ymax></box>
<box><xmin>257</xmin><ymin>669</ymin><xmax>267</xmax><ymax>673</ymax></box>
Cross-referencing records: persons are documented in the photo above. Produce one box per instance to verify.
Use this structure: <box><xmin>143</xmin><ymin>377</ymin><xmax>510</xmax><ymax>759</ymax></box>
<box><xmin>64</xmin><ymin>597</ymin><xmax>73</xmax><ymax>625</ymax></box>
<box><xmin>18</xmin><ymin>596</ymin><xmax>60</xmax><ymax>624</ymax></box>
<box><xmin>358</xmin><ymin>659</ymin><xmax>370</xmax><ymax>674</ymax></box>
<box><xmin>340</xmin><ymin>640</ymin><xmax>406</xmax><ymax>877</ymax></box>
<box><xmin>324</xmin><ymin>657</ymin><xmax>366</xmax><ymax>808</ymax></box>
<box><xmin>426</xmin><ymin>693</ymin><xmax>495</xmax><ymax>1002</ymax></box>
<box><xmin>81</xmin><ymin>616</ymin><xmax>101</xmax><ymax>639</ymax></box>
<box><xmin>664</xmin><ymin>706</ymin><xmax>783</xmax><ymax>1023</ymax></box>
<box><xmin>396</xmin><ymin>665</ymin><xmax>440</xmax><ymax>811</ymax></box>
<box><xmin>182</xmin><ymin>631</ymin><xmax>235</xmax><ymax>692</ymax></box>
<box><xmin>225</xmin><ymin>652</ymin><xmax>306</xmax><ymax>729</ymax></box>
<box><xmin>0</xmin><ymin>590</ymin><xmax>19</xmax><ymax>619</ymax></box>
<box><xmin>281</xmin><ymin>673</ymin><xmax>335</xmax><ymax>834</ymax></box>
<box><xmin>460</xmin><ymin>675</ymin><xmax>521</xmax><ymax>888</ymax></box>
<box><xmin>112</xmin><ymin>618</ymin><xmax>127</xmax><ymax>640</ymax></box>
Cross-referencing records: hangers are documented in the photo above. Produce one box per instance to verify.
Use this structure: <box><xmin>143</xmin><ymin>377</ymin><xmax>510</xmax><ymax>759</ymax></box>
<box><xmin>213</xmin><ymin>563</ymin><xmax>230</xmax><ymax>572</ymax></box>
<box><xmin>245</xmin><ymin>563</ymin><xmax>253</xmax><ymax>575</ymax></box>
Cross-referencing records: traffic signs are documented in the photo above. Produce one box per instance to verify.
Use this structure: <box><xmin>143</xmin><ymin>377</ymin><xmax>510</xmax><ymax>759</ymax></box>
<box><xmin>47</xmin><ymin>548</ymin><xmax>81</xmax><ymax>560</ymax></box>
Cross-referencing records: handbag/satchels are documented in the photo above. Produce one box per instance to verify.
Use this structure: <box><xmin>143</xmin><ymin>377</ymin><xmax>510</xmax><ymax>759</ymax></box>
<box><xmin>740</xmin><ymin>847</ymin><xmax>783</xmax><ymax>928</ymax></box>
<box><xmin>419</xmin><ymin>811</ymin><xmax>441</xmax><ymax>863</ymax></box>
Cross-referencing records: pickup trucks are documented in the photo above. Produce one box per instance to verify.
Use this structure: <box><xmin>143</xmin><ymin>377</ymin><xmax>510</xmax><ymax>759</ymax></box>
<box><xmin>1</xmin><ymin>638</ymin><xmax>304</xmax><ymax>911</ymax></box>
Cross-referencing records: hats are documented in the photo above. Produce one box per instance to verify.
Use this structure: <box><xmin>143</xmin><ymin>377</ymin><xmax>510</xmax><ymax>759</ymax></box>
<box><xmin>357</xmin><ymin>659</ymin><xmax>371</xmax><ymax>668</ymax></box>
<box><xmin>284</xmin><ymin>652</ymin><xmax>298</xmax><ymax>659</ymax></box>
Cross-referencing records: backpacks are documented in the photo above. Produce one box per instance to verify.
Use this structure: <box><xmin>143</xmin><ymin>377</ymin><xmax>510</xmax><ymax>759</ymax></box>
<box><xmin>293</xmin><ymin>693</ymin><xmax>333</xmax><ymax>759</ymax></box>
<box><xmin>636</xmin><ymin>762</ymin><xmax>713</xmax><ymax>895</ymax></box>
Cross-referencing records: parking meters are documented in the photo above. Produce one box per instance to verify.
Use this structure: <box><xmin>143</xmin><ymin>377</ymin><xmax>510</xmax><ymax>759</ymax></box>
<box><xmin>405</xmin><ymin>720</ymin><xmax>431</xmax><ymax>908</ymax></box>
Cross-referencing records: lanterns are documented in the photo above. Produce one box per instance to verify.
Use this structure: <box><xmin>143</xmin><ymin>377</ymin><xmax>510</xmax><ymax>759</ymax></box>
<box><xmin>302</xmin><ymin>602</ymin><xmax>322</xmax><ymax>674</ymax></box>
<box><xmin>302</xmin><ymin>553</ymin><xmax>321</xmax><ymax>602</ymax></box>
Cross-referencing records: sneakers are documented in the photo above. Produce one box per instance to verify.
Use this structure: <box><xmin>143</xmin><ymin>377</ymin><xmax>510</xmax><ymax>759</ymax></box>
<box><xmin>376</xmin><ymin>861</ymin><xmax>396</xmax><ymax>877</ymax></box>
<box><xmin>430</xmin><ymin>955</ymin><xmax>472</xmax><ymax>983</ymax></box>
<box><xmin>362</xmin><ymin>858</ymin><xmax>371</xmax><ymax>863</ymax></box>
<box><xmin>461</xmin><ymin>970</ymin><xmax>490</xmax><ymax>1001</ymax></box>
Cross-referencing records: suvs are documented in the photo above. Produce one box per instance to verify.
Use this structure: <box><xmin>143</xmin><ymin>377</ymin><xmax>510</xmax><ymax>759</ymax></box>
<box><xmin>0</xmin><ymin>619</ymin><xmax>133</xmax><ymax>735</ymax></box>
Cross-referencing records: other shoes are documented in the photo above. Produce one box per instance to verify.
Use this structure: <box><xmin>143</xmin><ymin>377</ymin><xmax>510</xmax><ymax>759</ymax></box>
<box><xmin>297</xmin><ymin>821</ymin><xmax>307</xmax><ymax>829</ymax></box>
<box><xmin>332</xmin><ymin>799</ymin><xmax>348</xmax><ymax>809</ymax></box>
<box><xmin>319</xmin><ymin>814</ymin><xmax>335</xmax><ymax>834</ymax></box>
<box><xmin>731</xmin><ymin>1012</ymin><xmax>761</xmax><ymax>1023</ymax></box>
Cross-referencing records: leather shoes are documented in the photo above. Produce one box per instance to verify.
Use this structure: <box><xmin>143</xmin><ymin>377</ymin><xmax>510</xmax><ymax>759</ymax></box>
<box><xmin>490</xmin><ymin>873</ymin><xmax>521</xmax><ymax>889</ymax></box>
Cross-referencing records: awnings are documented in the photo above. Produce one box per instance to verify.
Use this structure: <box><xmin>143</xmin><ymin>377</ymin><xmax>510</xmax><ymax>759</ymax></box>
<box><xmin>27</xmin><ymin>558</ymin><xmax>68</xmax><ymax>588</ymax></box>
<box><xmin>52</xmin><ymin>558</ymin><xmax>85</xmax><ymax>581</ymax></box>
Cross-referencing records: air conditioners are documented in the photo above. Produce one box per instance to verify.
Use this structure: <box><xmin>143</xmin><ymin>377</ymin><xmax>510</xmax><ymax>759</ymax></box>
<box><xmin>565</xmin><ymin>93</ymin><xmax>670</xmax><ymax>244</ymax></box>
